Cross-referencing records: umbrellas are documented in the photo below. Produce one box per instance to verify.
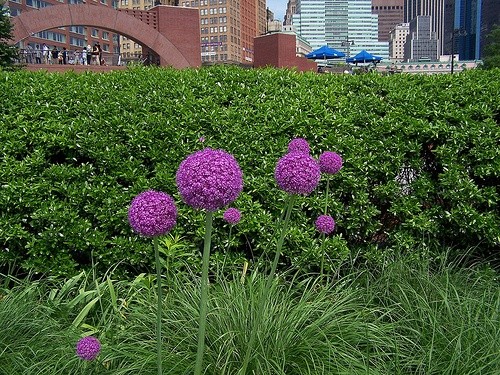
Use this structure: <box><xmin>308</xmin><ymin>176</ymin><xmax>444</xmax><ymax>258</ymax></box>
<box><xmin>346</xmin><ymin>50</ymin><xmax>382</xmax><ymax>67</ymax></box>
<box><xmin>305</xmin><ymin>45</ymin><xmax>345</xmax><ymax>65</ymax></box>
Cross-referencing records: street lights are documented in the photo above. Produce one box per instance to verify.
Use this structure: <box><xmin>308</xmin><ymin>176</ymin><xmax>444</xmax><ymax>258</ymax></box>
<box><xmin>451</xmin><ymin>25</ymin><xmax>467</xmax><ymax>74</ymax></box>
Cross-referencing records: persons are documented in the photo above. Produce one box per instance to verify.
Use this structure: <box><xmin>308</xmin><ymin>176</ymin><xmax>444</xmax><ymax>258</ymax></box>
<box><xmin>92</xmin><ymin>44</ymin><xmax>104</xmax><ymax>65</ymax></box>
<box><xmin>74</xmin><ymin>49</ymin><xmax>81</xmax><ymax>65</ymax></box>
<box><xmin>62</xmin><ymin>47</ymin><xmax>66</xmax><ymax>65</ymax></box>
<box><xmin>390</xmin><ymin>63</ymin><xmax>395</xmax><ymax>71</ymax></box>
<box><xmin>52</xmin><ymin>46</ymin><xmax>59</xmax><ymax>64</ymax></box>
<box><xmin>35</xmin><ymin>45</ymin><xmax>42</xmax><ymax>64</ymax></box>
<box><xmin>26</xmin><ymin>43</ymin><xmax>33</xmax><ymax>64</ymax></box>
<box><xmin>101</xmin><ymin>58</ymin><xmax>106</xmax><ymax>66</ymax></box>
<box><xmin>87</xmin><ymin>45</ymin><xmax>91</xmax><ymax>65</ymax></box>
<box><xmin>58</xmin><ymin>50</ymin><xmax>63</xmax><ymax>64</ymax></box>
<box><xmin>82</xmin><ymin>47</ymin><xmax>87</xmax><ymax>65</ymax></box>
<box><xmin>42</xmin><ymin>43</ymin><xmax>48</xmax><ymax>64</ymax></box>
<box><xmin>48</xmin><ymin>48</ymin><xmax>52</xmax><ymax>64</ymax></box>
<box><xmin>14</xmin><ymin>46</ymin><xmax>21</xmax><ymax>63</ymax></box>
<box><xmin>318</xmin><ymin>67</ymin><xmax>322</xmax><ymax>73</ymax></box>
